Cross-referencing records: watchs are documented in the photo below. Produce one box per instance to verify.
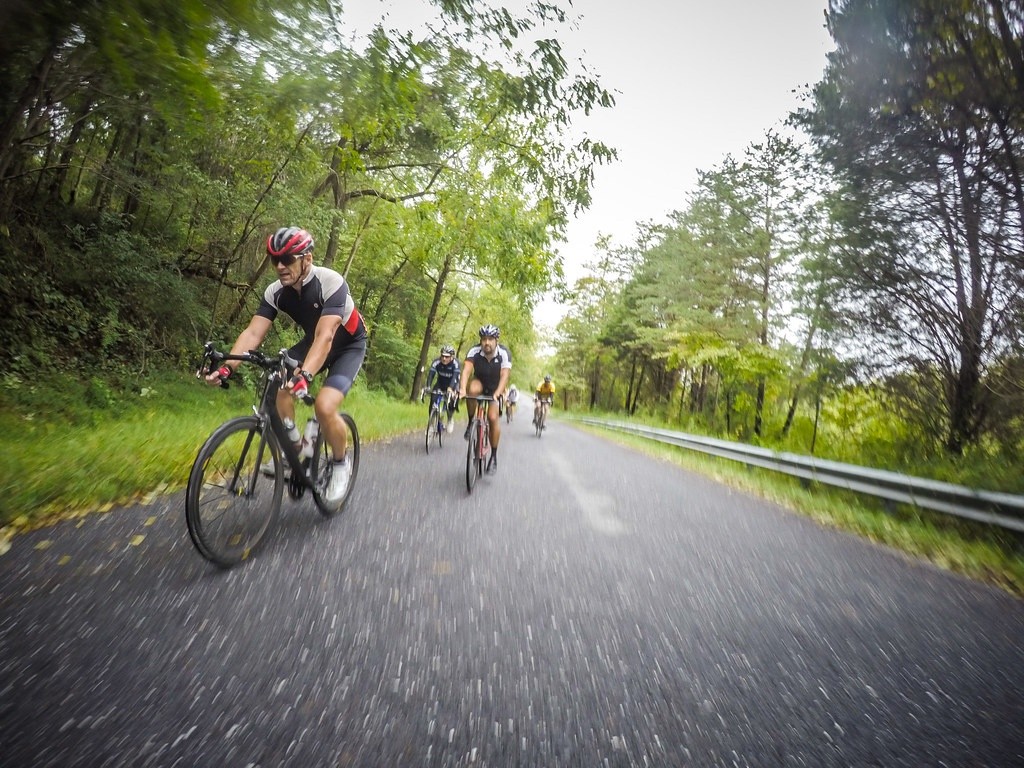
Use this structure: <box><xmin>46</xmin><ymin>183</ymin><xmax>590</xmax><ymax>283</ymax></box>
<box><xmin>298</xmin><ymin>370</ymin><xmax>313</xmax><ymax>384</ymax></box>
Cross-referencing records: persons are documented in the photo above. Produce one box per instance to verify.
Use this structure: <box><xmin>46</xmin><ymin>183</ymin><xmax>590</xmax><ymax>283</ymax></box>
<box><xmin>424</xmin><ymin>346</ymin><xmax>460</xmax><ymax>437</ymax></box>
<box><xmin>532</xmin><ymin>375</ymin><xmax>555</xmax><ymax>431</ymax></box>
<box><xmin>506</xmin><ymin>383</ymin><xmax>518</xmax><ymax>421</ymax></box>
<box><xmin>458</xmin><ymin>324</ymin><xmax>513</xmax><ymax>476</ymax></box>
<box><xmin>195</xmin><ymin>227</ymin><xmax>367</xmax><ymax>502</ymax></box>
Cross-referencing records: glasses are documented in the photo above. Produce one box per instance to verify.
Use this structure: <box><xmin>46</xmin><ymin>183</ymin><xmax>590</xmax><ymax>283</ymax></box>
<box><xmin>441</xmin><ymin>354</ymin><xmax>450</xmax><ymax>359</ymax></box>
<box><xmin>271</xmin><ymin>254</ymin><xmax>307</xmax><ymax>266</ymax></box>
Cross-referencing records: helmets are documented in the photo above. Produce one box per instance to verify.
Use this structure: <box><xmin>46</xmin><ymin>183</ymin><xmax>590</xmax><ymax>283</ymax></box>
<box><xmin>544</xmin><ymin>375</ymin><xmax>551</xmax><ymax>381</ymax></box>
<box><xmin>441</xmin><ymin>346</ymin><xmax>455</xmax><ymax>355</ymax></box>
<box><xmin>267</xmin><ymin>227</ymin><xmax>315</xmax><ymax>255</ymax></box>
<box><xmin>479</xmin><ymin>324</ymin><xmax>500</xmax><ymax>338</ymax></box>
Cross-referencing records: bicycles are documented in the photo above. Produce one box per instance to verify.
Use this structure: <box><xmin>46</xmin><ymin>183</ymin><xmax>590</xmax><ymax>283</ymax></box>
<box><xmin>454</xmin><ymin>390</ymin><xmax>503</xmax><ymax>493</ymax></box>
<box><xmin>535</xmin><ymin>398</ymin><xmax>554</xmax><ymax>438</ymax></box>
<box><xmin>421</xmin><ymin>388</ymin><xmax>451</xmax><ymax>454</ymax></box>
<box><xmin>185</xmin><ymin>341</ymin><xmax>359</xmax><ymax>568</ymax></box>
<box><xmin>504</xmin><ymin>399</ymin><xmax>515</xmax><ymax>424</ymax></box>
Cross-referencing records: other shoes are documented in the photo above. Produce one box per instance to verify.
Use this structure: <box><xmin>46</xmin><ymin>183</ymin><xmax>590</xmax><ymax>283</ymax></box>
<box><xmin>424</xmin><ymin>426</ymin><xmax>434</xmax><ymax>436</ymax></box>
<box><xmin>487</xmin><ymin>456</ymin><xmax>497</xmax><ymax>474</ymax></box>
<box><xmin>260</xmin><ymin>445</ymin><xmax>304</xmax><ymax>478</ymax></box>
<box><xmin>326</xmin><ymin>455</ymin><xmax>351</xmax><ymax>502</ymax></box>
<box><xmin>447</xmin><ymin>418</ymin><xmax>454</xmax><ymax>434</ymax></box>
<box><xmin>464</xmin><ymin>422</ymin><xmax>473</xmax><ymax>440</ymax></box>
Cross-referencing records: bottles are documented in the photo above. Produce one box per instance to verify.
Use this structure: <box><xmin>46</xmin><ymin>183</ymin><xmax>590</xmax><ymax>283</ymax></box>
<box><xmin>302</xmin><ymin>416</ymin><xmax>320</xmax><ymax>459</ymax></box>
<box><xmin>284</xmin><ymin>416</ymin><xmax>301</xmax><ymax>454</ymax></box>
